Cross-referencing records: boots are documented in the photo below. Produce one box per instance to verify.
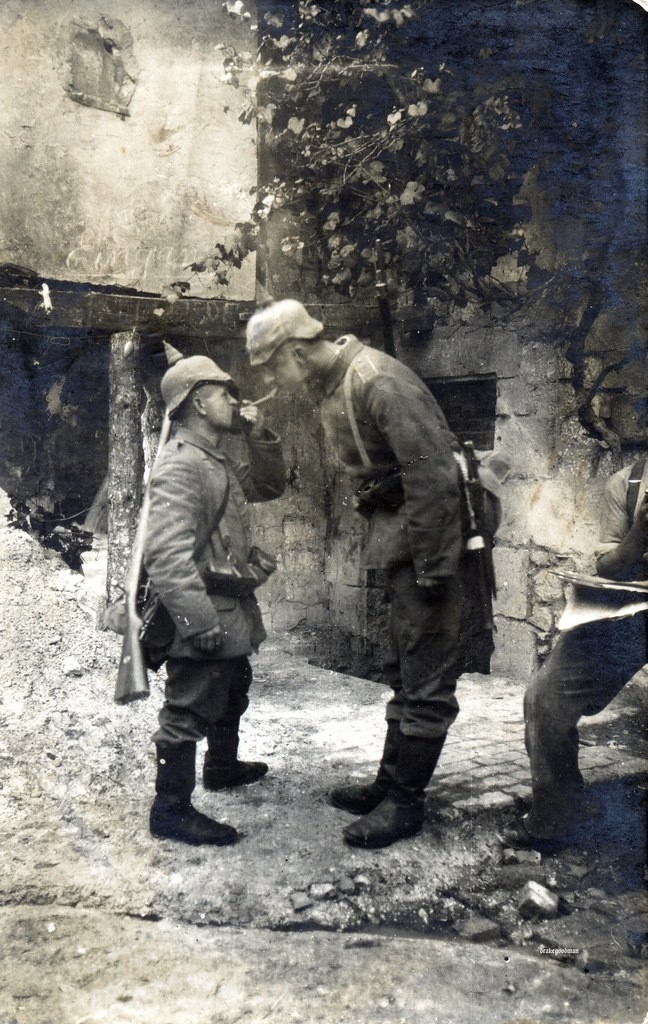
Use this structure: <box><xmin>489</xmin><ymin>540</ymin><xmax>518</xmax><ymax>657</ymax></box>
<box><xmin>203</xmin><ymin>716</ymin><xmax>269</xmax><ymax>790</ymax></box>
<box><xmin>342</xmin><ymin>734</ymin><xmax>447</xmax><ymax>849</ymax></box>
<box><xmin>329</xmin><ymin>720</ymin><xmax>400</xmax><ymax>815</ymax></box>
<box><xmin>149</xmin><ymin>739</ymin><xmax>238</xmax><ymax>846</ymax></box>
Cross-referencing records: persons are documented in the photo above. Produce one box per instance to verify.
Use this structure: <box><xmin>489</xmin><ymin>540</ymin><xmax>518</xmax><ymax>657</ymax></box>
<box><xmin>496</xmin><ymin>455</ymin><xmax>648</xmax><ymax>851</ymax></box>
<box><xmin>245</xmin><ymin>297</ymin><xmax>473</xmax><ymax>852</ymax></box>
<box><xmin>138</xmin><ymin>353</ymin><xmax>288</xmax><ymax>844</ymax></box>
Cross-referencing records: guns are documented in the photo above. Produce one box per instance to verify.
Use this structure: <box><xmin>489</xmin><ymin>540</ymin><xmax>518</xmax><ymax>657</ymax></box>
<box><xmin>113</xmin><ymin>405</ymin><xmax>170</xmax><ymax>704</ymax></box>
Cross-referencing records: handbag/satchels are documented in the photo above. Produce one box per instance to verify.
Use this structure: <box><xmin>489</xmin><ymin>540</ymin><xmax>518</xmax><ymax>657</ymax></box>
<box><xmin>139</xmin><ymin>591</ymin><xmax>177</xmax><ymax>672</ymax></box>
<box><xmin>453</xmin><ymin>450</ymin><xmax>503</xmax><ymax>534</ymax></box>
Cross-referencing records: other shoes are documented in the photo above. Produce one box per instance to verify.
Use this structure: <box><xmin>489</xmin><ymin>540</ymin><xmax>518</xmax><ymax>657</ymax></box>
<box><xmin>495</xmin><ymin>820</ymin><xmax>545</xmax><ymax>850</ymax></box>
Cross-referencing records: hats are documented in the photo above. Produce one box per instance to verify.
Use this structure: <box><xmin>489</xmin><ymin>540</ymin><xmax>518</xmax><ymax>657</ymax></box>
<box><xmin>244</xmin><ymin>299</ymin><xmax>324</xmax><ymax>367</ymax></box>
<box><xmin>159</xmin><ymin>354</ymin><xmax>239</xmax><ymax>420</ymax></box>
